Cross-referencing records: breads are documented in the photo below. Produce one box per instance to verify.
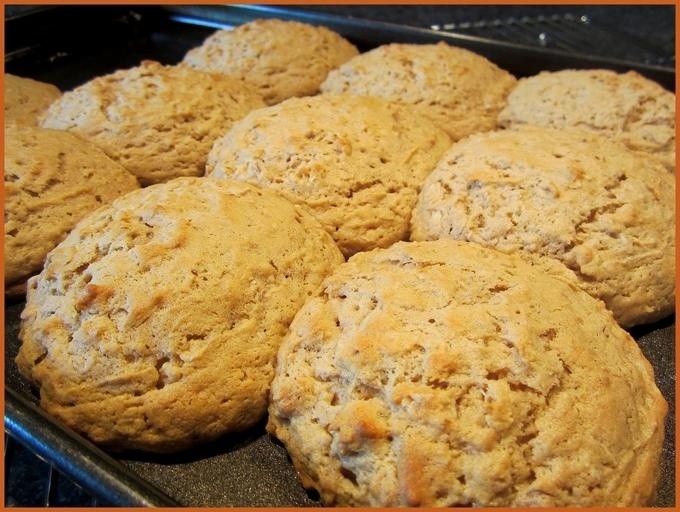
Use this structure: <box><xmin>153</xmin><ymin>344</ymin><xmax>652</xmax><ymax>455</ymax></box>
<box><xmin>4</xmin><ymin>17</ymin><xmax>676</xmax><ymax>507</ymax></box>
